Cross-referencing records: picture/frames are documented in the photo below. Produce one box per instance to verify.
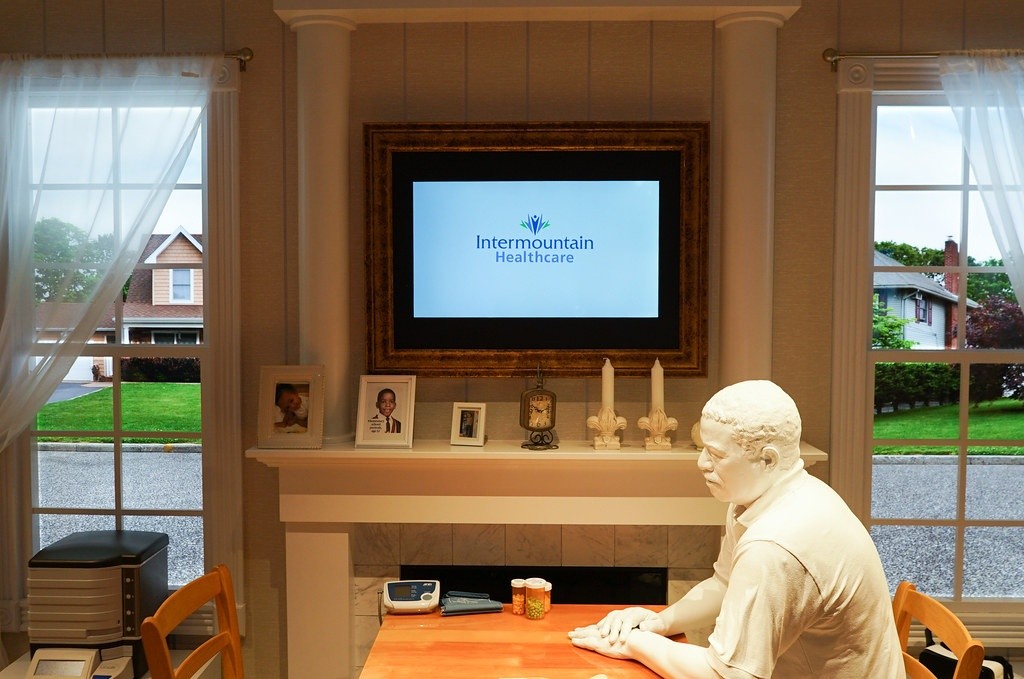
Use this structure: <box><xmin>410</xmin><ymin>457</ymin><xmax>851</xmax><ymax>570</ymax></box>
<box><xmin>257</xmin><ymin>366</ymin><xmax>324</xmax><ymax>449</ymax></box>
<box><xmin>451</xmin><ymin>402</ymin><xmax>486</xmax><ymax>447</ymax></box>
<box><xmin>355</xmin><ymin>374</ymin><xmax>416</xmax><ymax>449</ymax></box>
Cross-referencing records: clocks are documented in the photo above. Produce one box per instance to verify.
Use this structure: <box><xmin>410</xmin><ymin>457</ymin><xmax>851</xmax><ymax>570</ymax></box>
<box><xmin>519</xmin><ymin>364</ymin><xmax>559</xmax><ymax>451</ymax></box>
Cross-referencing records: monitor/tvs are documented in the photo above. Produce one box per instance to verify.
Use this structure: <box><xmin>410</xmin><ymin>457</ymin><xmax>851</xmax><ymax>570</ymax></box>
<box><xmin>389</xmin><ymin>149</ymin><xmax>681</xmax><ymax>351</ymax></box>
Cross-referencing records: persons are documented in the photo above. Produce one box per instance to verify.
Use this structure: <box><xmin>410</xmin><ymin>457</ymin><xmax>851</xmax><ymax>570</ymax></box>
<box><xmin>567</xmin><ymin>379</ymin><xmax>906</xmax><ymax>679</ymax></box>
<box><xmin>465</xmin><ymin>413</ymin><xmax>473</xmax><ymax>436</ymax></box>
<box><xmin>370</xmin><ymin>388</ymin><xmax>401</xmax><ymax>433</ymax></box>
<box><xmin>274</xmin><ymin>383</ymin><xmax>308</xmax><ymax>428</ymax></box>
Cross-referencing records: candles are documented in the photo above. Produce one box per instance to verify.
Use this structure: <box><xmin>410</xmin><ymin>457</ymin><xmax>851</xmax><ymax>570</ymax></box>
<box><xmin>602</xmin><ymin>358</ymin><xmax>614</xmax><ymax>414</ymax></box>
<box><xmin>651</xmin><ymin>357</ymin><xmax>665</xmax><ymax>412</ymax></box>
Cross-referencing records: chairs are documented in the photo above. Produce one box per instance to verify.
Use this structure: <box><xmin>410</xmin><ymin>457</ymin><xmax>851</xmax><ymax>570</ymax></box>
<box><xmin>141</xmin><ymin>564</ymin><xmax>244</xmax><ymax>679</ymax></box>
<box><xmin>892</xmin><ymin>582</ymin><xmax>985</xmax><ymax>679</ymax></box>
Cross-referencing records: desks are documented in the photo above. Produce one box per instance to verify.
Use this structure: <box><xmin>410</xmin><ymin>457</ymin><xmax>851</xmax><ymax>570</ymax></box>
<box><xmin>358</xmin><ymin>603</ymin><xmax>690</xmax><ymax>679</ymax></box>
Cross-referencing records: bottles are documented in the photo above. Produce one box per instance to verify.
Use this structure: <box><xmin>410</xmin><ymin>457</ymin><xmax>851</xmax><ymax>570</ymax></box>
<box><xmin>525</xmin><ymin>578</ymin><xmax>546</xmax><ymax>620</ymax></box>
<box><xmin>545</xmin><ymin>582</ymin><xmax>552</xmax><ymax>613</ymax></box>
<box><xmin>511</xmin><ymin>578</ymin><xmax>526</xmax><ymax>615</ymax></box>
